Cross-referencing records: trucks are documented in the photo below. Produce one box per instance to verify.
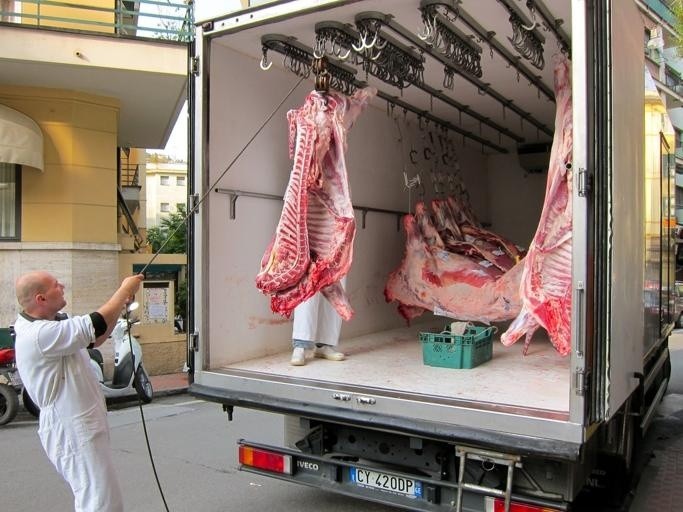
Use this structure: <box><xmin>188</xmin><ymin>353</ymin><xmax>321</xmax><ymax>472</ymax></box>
<box><xmin>183</xmin><ymin>1</ymin><xmax>680</xmax><ymax>511</ymax></box>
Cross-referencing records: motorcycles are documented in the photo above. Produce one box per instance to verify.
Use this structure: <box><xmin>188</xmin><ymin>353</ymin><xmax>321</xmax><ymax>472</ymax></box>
<box><xmin>0</xmin><ymin>327</ymin><xmax>21</xmax><ymax>426</ymax></box>
<box><xmin>23</xmin><ymin>302</ymin><xmax>153</xmax><ymax>418</ymax></box>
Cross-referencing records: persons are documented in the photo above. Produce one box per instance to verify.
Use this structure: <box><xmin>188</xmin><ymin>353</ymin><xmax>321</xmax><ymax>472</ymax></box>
<box><xmin>13</xmin><ymin>269</ymin><xmax>145</xmax><ymax>512</ymax></box>
<box><xmin>287</xmin><ymin>275</ymin><xmax>348</xmax><ymax>366</ymax></box>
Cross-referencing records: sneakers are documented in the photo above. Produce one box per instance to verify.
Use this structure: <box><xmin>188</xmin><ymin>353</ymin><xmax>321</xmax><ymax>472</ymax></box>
<box><xmin>313</xmin><ymin>345</ymin><xmax>344</xmax><ymax>360</ymax></box>
<box><xmin>292</xmin><ymin>348</ymin><xmax>305</xmax><ymax>365</ymax></box>
